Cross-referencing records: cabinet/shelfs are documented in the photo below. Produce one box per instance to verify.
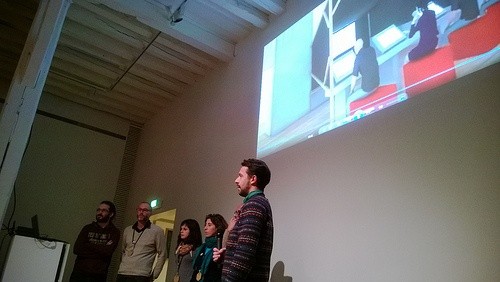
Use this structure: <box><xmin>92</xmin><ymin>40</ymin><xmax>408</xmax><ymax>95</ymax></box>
<box><xmin>0</xmin><ymin>235</ymin><xmax>70</xmax><ymax>282</ymax></box>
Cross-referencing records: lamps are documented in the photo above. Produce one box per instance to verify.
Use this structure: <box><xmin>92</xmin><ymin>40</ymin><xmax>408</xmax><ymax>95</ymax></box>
<box><xmin>172</xmin><ymin>9</ymin><xmax>183</xmax><ymax>22</ymax></box>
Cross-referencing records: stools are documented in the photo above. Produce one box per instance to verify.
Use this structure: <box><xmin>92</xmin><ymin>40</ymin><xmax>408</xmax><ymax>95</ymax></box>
<box><xmin>448</xmin><ymin>1</ymin><xmax>500</xmax><ymax>59</ymax></box>
<box><xmin>404</xmin><ymin>46</ymin><xmax>459</xmax><ymax>97</ymax></box>
<box><xmin>350</xmin><ymin>84</ymin><xmax>398</xmax><ymax>115</ymax></box>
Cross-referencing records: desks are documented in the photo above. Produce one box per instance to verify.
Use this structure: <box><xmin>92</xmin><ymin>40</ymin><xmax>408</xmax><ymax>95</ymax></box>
<box><xmin>325</xmin><ymin>0</ymin><xmax>496</xmax><ymax>97</ymax></box>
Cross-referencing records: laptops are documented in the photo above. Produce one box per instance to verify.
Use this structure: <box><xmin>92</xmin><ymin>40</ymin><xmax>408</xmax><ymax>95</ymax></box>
<box><xmin>32</xmin><ymin>214</ymin><xmax>57</xmax><ymax>241</ymax></box>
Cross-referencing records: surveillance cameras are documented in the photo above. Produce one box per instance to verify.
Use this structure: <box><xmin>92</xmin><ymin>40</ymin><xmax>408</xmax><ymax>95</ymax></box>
<box><xmin>173</xmin><ymin>13</ymin><xmax>184</xmax><ymax>23</ymax></box>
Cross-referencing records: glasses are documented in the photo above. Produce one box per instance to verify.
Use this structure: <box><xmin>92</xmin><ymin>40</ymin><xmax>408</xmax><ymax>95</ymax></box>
<box><xmin>137</xmin><ymin>208</ymin><xmax>150</xmax><ymax>212</ymax></box>
<box><xmin>96</xmin><ymin>208</ymin><xmax>109</xmax><ymax>213</ymax></box>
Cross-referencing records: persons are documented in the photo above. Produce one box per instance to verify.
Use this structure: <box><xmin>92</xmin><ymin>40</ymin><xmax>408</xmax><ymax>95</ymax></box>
<box><xmin>69</xmin><ymin>201</ymin><xmax>121</xmax><ymax>282</ymax></box>
<box><xmin>174</xmin><ymin>219</ymin><xmax>202</xmax><ymax>282</ymax></box>
<box><xmin>346</xmin><ymin>39</ymin><xmax>380</xmax><ymax>117</ymax></box>
<box><xmin>190</xmin><ymin>214</ymin><xmax>228</xmax><ymax>282</ymax></box>
<box><xmin>213</xmin><ymin>158</ymin><xmax>273</xmax><ymax>282</ymax></box>
<box><xmin>444</xmin><ymin>0</ymin><xmax>479</xmax><ymax>43</ymax></box>
<box><xmin>405</xmin><ymin>0</ymin><xmax>439</xmax><ymax>62</ymax></box>
<box><xmin>116</xmin><ymin>202</ymin><xmax>167</xmax><ymax>282</ymax></box>
